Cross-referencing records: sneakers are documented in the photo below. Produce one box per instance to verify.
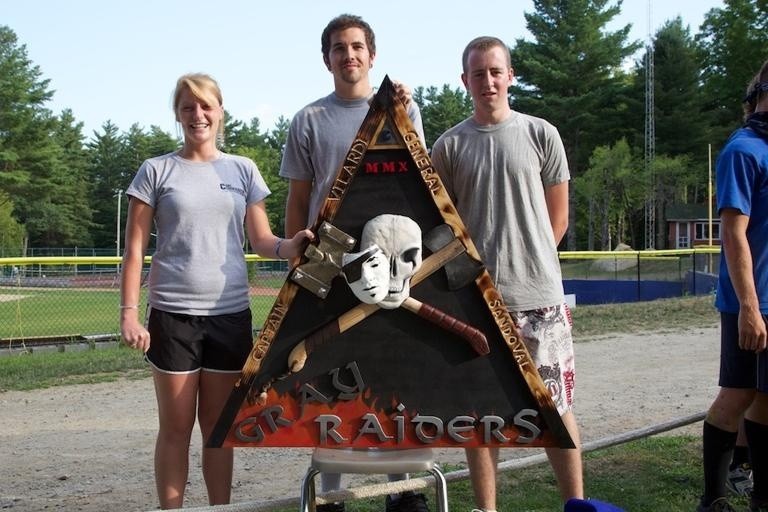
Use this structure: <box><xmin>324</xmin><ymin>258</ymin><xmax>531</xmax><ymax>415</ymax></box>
<box><xmin>315</xmin><ymin>500</ymin><xmax>344</xmax><ymax>512</ymax></box>
<box><xmin>724</xmin><ymin>463</ymin><xmax>754</xmax><ymax>503</ymax></box>
<box><xmin>695</xmin><ymin>493</ymin><xmax>736</xmax><ymax>512</ymax></box>
<box><xmin>384</xmin><ymin>492</ymin><xmax>430</xmax><ymax>512</ymax></box>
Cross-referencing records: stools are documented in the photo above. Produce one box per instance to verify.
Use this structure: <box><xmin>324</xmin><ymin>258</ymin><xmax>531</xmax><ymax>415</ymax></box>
<box><xmin>298</xmin><ymin>447</ymin><xmax>449</xmax><ymax>512</ymax></box>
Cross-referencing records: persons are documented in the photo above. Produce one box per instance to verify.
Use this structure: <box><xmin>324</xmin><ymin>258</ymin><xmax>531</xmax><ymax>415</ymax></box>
<box><xmin>118</xmin><ymin>73</ymin><xmax>315</xmax><ymax>511</ymax></box>
<box><xmin>697</xmin><ymin>62</ymin><xmax>768</xmax><ymax>511</ymax></box>
<box><xmin>429</xmin><ymin>34</ymin><xmax>587</xmax><ymax>512</ymax></box>
<box><xmin>724</xmin><ymin>413</ymin><xmax>755</xmax><ymax>499</ymax></box>
<box><xmin>276</xmin><ymin>12</ymin><xmax>435</xmax><ymax>511</ymax></box>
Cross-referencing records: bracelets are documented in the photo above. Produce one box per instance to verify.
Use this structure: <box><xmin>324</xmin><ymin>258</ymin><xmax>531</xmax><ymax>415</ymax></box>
<box><xmin>118</xmin><ymin>303</ymin><xmax>139</xmax><ymax>308</ymax></box>
<box><xmin>275</xmin><ymin>237</ymin><xmax>285</xmax><ymax>260</ymax></box>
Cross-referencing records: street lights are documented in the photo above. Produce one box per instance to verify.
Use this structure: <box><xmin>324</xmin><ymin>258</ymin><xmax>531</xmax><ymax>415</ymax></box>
<box><xmin>111</xmin><ymin>187</ymin><xmax>124</xmax><ymax>272</ymax></box>
